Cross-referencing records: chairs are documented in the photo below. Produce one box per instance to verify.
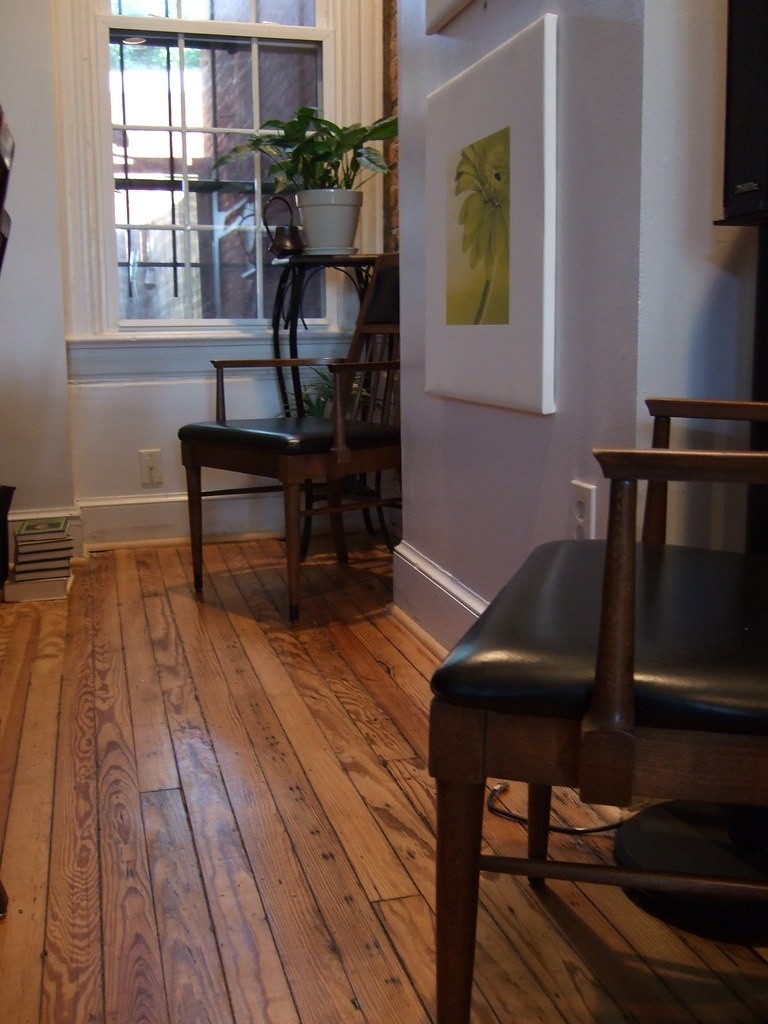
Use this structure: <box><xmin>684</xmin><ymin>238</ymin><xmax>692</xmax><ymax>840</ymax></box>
<box><xmin>179</xmin><ymin>252</ymin><xmax>403</xmax><ymax>625</ymax></box>
<box><xmin>428</xmin><ymin>396</ymin><xmax>768</xmax><ymax>1024</ymax></box>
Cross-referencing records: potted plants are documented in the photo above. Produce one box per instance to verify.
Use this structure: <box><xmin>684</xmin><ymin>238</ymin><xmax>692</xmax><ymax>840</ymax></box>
<box><xmin>212</xmin><ymin>106</ymin><xmax>397</xmax><ymax>252</ymax></box>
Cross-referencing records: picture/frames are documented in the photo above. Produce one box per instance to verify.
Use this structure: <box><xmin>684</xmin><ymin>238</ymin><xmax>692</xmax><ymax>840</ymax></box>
<box><xmin>424</xmin><ymin>11</ymin><xmax>559</xmax><ymax>415</ymax></box>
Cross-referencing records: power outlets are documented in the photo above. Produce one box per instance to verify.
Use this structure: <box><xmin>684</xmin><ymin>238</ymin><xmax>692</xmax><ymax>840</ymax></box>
<box><xmin>139</xmin><ymin>449</ymin><xmax>164</xmax><ymax>485</ymax></box>
<box><xmin>569</xmin><ymin>480</ymin><xmax>597</xmax><ymax>541</ymax></box>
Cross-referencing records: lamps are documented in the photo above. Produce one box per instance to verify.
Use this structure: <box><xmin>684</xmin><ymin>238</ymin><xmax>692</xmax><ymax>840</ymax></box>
<box><xmin>262</xmin><ymin>197</ymin><xmax>301</xmax><ymax>258</ymax></box>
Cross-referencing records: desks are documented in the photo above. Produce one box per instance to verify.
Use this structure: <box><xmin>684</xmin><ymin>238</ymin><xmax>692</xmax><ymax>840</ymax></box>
<box><xmin>272</xmin><ymin>254</ymin><xmax>393</xmax><ymax>565</ymax></box>
